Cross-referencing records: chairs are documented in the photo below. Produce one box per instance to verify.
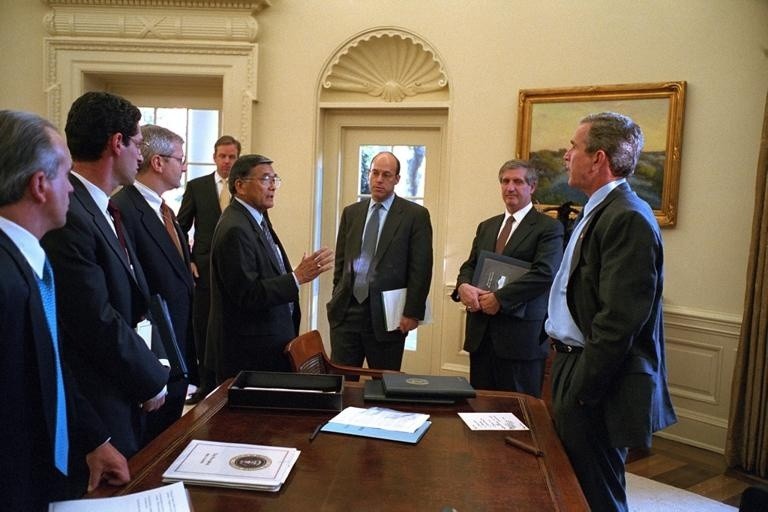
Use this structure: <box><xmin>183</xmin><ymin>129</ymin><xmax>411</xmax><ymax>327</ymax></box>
<box><xmin>283</xmin><ymin>331</ymin><xmax>406</xmax><ymax>381</ymax></box>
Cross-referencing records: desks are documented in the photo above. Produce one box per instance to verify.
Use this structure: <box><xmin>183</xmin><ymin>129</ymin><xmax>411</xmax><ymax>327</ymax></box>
<box><xmin>83</xmin><ymin>375</ymin><xmax>592</xmax><ymax>512</ymax></box>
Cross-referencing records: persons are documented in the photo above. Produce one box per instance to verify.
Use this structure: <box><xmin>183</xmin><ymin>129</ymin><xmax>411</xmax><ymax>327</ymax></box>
<box><xmin>326</xmin><ymin>152</ymin><xmax>433</xmax><ymax>381</ymax></box>
<box><xmin>108</xmin><ymin>125</ymin><xmax>194</xmax><ymax>438</ymax></box>
<box><xmin>177</xmin><ymin>135</ymin><xmax>243</xmax><ymax>405</ymax></box>
<box><xmin>205</xmin><ymin>155</ymin><xmax>334</xmax><ymax>385</ymax></box>
<box><xmin>40</xmin><ymin>91</ymin><xmax>169</xmax><ymax>479</ymax></box>
<box><xmin>545</xmin><ymin>112</ymin><xmax>678</xmax><ymax>512</ymax></box>
<box><xmin>0</xmin><ymin>110</ymin><xmax>130</xmax><ymax>512</ymax></box>
<box><xmin>450</xmin><ymin>158</ymin><xmax>565</xmax><ymax>397</ymax></box>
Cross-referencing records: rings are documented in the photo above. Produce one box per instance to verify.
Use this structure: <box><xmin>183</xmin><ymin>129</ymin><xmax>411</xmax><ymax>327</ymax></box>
<box><xmin>468</xmin><ymin>306</ymin><xmax>471</xmax><ymax>309</ymax></box>
<box><xmin>317</xmin><ymin>263</ymin><xmax>320</xmax><ymax>268</ymax></box>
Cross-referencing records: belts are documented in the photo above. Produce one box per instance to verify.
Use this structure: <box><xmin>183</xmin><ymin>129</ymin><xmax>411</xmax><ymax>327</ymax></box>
<box><xmin>550</xmin><ymin>342</ymin><xmax>582</xmax><ymax>356</ymax></box>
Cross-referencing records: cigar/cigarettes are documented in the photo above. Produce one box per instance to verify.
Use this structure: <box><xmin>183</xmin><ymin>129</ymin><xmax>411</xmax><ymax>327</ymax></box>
<box><xmin>505</xmin><ymin>435</ymin><xmax>544</xmax><ymax>457</ymax></box>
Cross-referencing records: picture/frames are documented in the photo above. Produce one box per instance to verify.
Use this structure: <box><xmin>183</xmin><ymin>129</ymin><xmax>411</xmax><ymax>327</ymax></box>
<box><xmin>514</xmin><ymin>79</ymin><xmax>687</xmax><ymax>231</ymax></box>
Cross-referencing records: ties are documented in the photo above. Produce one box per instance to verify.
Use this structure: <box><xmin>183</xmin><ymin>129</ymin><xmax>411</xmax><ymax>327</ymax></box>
<box><xmin>33</xmin><ymin>255</ymin><xmax>70</xmax><ymax>478</ymax></box>
<box><xmin>107</xmin><ymin>200</ymin><xmax>129</xmax><ymax>258</ymax></box>
<box><xmin>220</xmin><ymin>179</ymin><xmax>229</xmax><ymax>212</ymax></box>
<box><xmin>161</xmin><ymin>201</ymin><xmax>182</xmax><ymax>260</ymax></box>
<box><xmin>260</xmin><ymin>219</ymin><xmax>294</xmax><ymax>313</ymax></box>
<box><xmin>495</xmin><ymin>216</ymin><xmax>515</xmax><ymax>256</ymax></box>
<box><xmin>352</xmin><ymin>203</ymin><xmax>382</xmax><ymax>305</ymax></box>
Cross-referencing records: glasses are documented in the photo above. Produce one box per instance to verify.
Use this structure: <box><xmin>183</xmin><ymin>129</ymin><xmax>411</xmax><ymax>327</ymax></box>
<box><xmin>128</xmin><ymin>137</ymin><xmax>150</xmax><ymax>155</ymax></box>
<box><xmin>160</xmin><ymin>155</ymin><xmax>187</xmax><ymax>165</ymax></box>
<box><xmin>243</xmin><ymin>176</ymin><xmax>282</xmax><ymax>189</ymax></box>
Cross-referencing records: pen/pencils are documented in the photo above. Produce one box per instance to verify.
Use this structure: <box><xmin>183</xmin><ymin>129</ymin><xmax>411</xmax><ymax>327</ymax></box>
<box><xmin>503</xmin><ymin>436</ymin><xmax>546</xmax><ymax>458</ymax></box>
<box><xmin>309</xmin><ymin>416</ymin><xmax>332</xmax><ymax>441</ymax></box>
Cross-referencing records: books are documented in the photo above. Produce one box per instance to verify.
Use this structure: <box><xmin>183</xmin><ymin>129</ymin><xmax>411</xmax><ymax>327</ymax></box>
<box><xmin>381</xmin><ymin>373</ymin><xmax>477</xmax><ymax>397</ymax></box>
<box><xmin>363</xmin><ymin>379</ymin><xmax>456</xmax><ymax>406</ymax></box>
<box><xmin>471</xmin><ymin>250</ymin><xmax>532</xmax><ymax>318</ymax></box>
